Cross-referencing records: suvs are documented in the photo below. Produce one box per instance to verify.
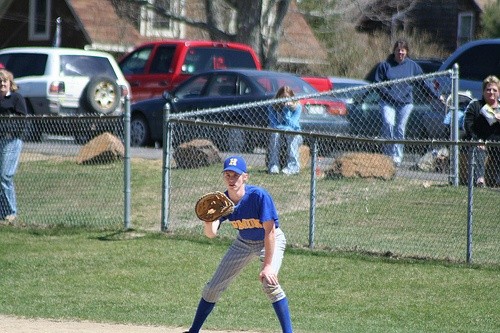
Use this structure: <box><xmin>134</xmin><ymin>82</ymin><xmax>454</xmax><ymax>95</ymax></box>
<box><xmin>0</xmin><ymin>47</ymin><xmax>132</xmax><ymax>145</ymax></box>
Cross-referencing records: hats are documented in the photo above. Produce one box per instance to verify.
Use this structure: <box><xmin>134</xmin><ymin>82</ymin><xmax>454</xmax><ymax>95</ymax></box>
<box><xmin>221</xmin><ymin>156</ymin><xmax>247</xmax><ymax>175</ymax></box>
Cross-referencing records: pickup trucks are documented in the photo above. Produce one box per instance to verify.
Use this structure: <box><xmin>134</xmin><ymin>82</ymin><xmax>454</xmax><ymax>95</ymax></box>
<box><xmin>115</xmin><ymin>39</ymin><xmax>347</xmax><ymax>117</ymax></box>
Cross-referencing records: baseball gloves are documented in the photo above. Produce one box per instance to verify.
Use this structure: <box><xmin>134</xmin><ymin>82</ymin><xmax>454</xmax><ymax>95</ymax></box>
<box><xmin>194</xmin><ymin>190</ymin><xmax>235</xmax><ymax>222</ymax></box>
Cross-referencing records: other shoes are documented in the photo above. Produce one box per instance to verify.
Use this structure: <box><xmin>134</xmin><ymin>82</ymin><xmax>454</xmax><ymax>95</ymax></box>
<box><xmin>0</xmin><ymin>213</ymin><xmax>17</xmax><ymax>226</ymax></box>
<box><xmin>476</xmin><ymin>177</ymin><xmax>485</xmax><ymax>188</ymax></box>
<box><xmin>281</xmin><ymin>167</ymin><xmax>299</xmax><ymax>174</ymax></box>
<box><xmin>269</xmin><ymin>165</ymin><xmax>279</xmax><ymax>174</ymax></box>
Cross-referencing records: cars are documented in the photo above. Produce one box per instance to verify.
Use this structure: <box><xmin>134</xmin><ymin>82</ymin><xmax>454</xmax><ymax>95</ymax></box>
<box><xmin>119</xmin><ymin>69</ymin><xmax>355</xmax><ymax>158</ymax></box>
<box><xmin>327</xmin><ymin>38</ymin><xmax>500</xmax><ymax>155</ymax></box>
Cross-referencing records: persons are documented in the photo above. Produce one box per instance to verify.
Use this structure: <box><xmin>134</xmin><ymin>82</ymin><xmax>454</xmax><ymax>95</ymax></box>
<box><xmin>0</xmin><ymin>69</ymin><xmax>27</xmax><ymax>227</ymax></box>
<box><xmin>267</xmin><ymin>85</ymin><xmax>303</xmax><ymax>175</ymax></box>
<box><xmin>182</xmin><ymin>156</ymin><xmax>292</xmax><ymax>333</ymax></box>
<box><xmin>373</xmin><ymin>37</ymin><xmax>446</xmax><ymax>171</ymax></box>
<box><xmin>462</xmin><ymin>75</ymin><xmax>500</xmax><ymax>189</ymax></box>
